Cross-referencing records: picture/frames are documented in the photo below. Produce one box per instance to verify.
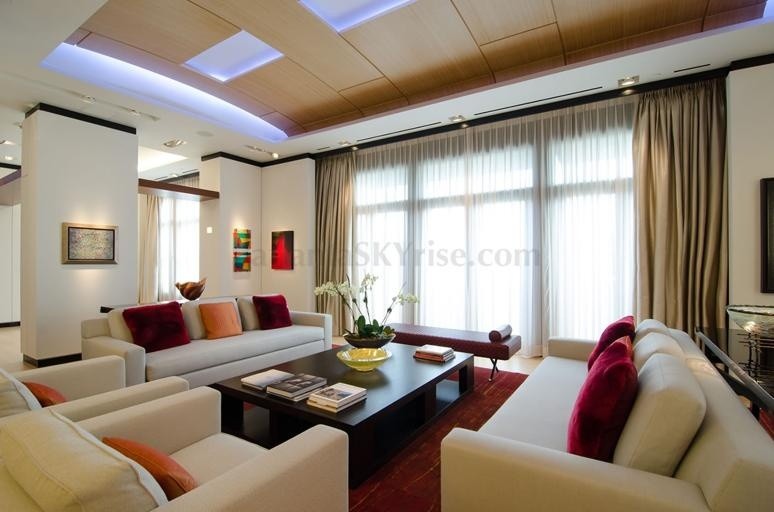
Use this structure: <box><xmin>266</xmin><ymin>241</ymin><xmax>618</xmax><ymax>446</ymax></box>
<box><xmin>60</xmin><ymin>222</ymin><xmax>121</xmax><ymax>265</ymax></box>
<box><xmin>760</xmin><ymin>177</ymin><xmax>774</xmax><ymax>295</ymax></box>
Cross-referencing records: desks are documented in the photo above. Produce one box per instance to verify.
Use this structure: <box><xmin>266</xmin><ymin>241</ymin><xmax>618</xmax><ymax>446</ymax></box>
<box><xmin>214</xmin><ymin>342</ymin><xmax>475</xmax><ymax>491</ymax></box>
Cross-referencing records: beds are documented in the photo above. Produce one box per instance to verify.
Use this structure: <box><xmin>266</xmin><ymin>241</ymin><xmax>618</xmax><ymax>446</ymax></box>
<box><xmin>389</xmin><ymin>322</ymin><xmax>522</xmax><ymax>380</ymax></box>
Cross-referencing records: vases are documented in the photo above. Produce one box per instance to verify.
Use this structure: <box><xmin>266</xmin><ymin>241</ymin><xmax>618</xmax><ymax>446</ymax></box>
<box><xmin>343</xmin><ymin>334</ymin><xmax>396</xmax><ymax>348</ymax></box>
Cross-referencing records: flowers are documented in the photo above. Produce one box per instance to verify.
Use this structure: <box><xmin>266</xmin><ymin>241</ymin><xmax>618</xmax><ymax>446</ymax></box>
<box><xmin>315</xmin><ymin>273</ymin><xmax>420</xmax><ymax>334</ymax></box>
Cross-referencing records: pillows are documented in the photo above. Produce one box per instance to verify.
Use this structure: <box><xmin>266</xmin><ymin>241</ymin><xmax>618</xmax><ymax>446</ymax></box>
<box><xmin>567</xmin><ymin>336</ymin><xmax>638</xmax><ymax>463</ymax></box>
<box><xmin>489</xmin><ymin>324</ymin><xmax>512</xmax><ymax>343</ymax></box>
<box><xmin>587</xmin><ymin>315</ymin><xmax>636</xmax><ymax>368</ymax></box>
<box><xmin>0</xmin><ymin>369</ymin><xmax>197</xmax><ymax>512</ymax></box>
<box><xmin>614</xmin><ymin>319</ymin><xmax>708</xmax><ymax>477</ymax></box>
<box><xmin>182</xmin><ymin>295</ymin><xmax>243</xmax><ymax>340</ymax></box>
<box><xmin>237</xmin><ymin>295</ymin><xmax>260</xmax><ymax>331</ymax></box>
<box><xmin>198</xmin><ymin>303</ymin><xmax>242</xmax><ymax>340</ymax></box>
<box><xmin>123</xmin><ymin>302</ymin><xmax>190</xmax><ymax>352</ymax></box>
<box><xmin>107</xmin><ymin>304</ymin><xmax>133</xmax><ymax>344</ymax></box>
<box><xmin>252</xmin><ymin>294</ymin><xmax>292</xmax><ymax>329</ymax></box>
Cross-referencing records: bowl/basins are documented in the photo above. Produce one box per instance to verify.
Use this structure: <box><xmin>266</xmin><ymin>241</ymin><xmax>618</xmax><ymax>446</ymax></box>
<box><xmin>336</xmin><ymin>348</ymin><xmax>392</xmax><ymax>372</ymax></box>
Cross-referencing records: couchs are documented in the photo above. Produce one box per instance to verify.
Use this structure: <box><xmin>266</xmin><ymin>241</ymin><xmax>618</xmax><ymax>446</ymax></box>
<box><xmin>0</xmin><ymin>356</ymin><xmax>188</xmax><ymax>423</ymax></box>
<box><xmin>440</xmin><ymin>318</ymin><xmax>774</xmax><ymax>512</ymax></box>
<box><xmin>1</xmin><ymin>438</ymin><xmax>349</xmax><ymax>512</ymax></box>
<box><xmin>80</xmin><ymin>295</ymin><xmax>333</xmax><ymax>388</ymax></box>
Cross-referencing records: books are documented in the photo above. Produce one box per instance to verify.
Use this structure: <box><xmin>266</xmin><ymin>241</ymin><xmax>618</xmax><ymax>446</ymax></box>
<box><xmin>241</xmin><ymin>369</ymin><xmax>367</xmax><ymax>414</ymax></box>
<box><xmin>413</xmin><ymin>344</ymin><xmax>455</xmax><ymax>362</ymax></box>
